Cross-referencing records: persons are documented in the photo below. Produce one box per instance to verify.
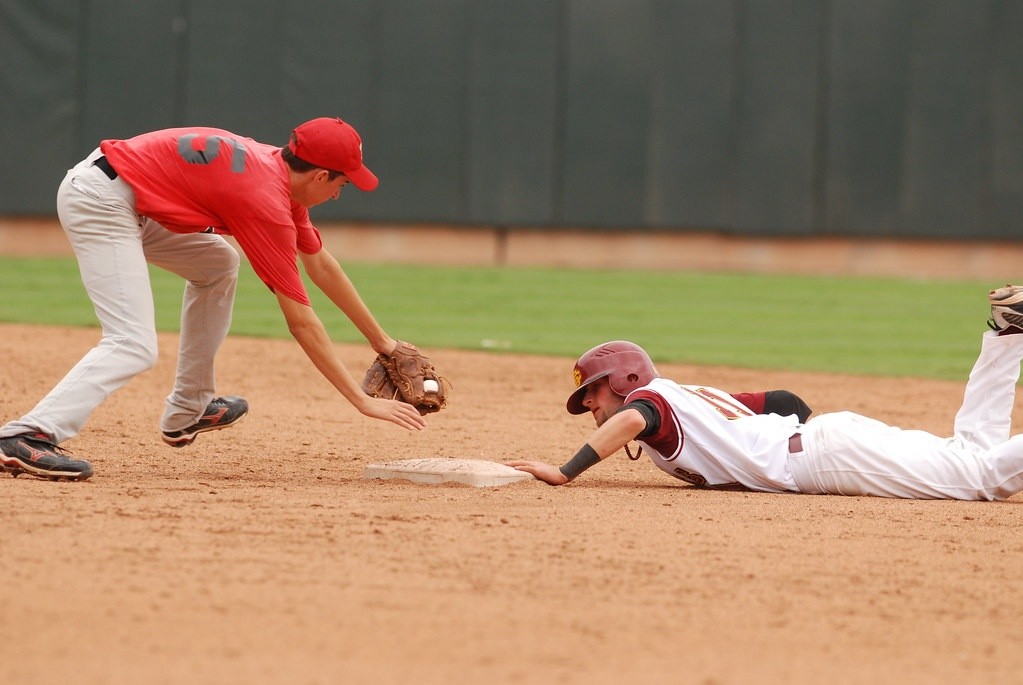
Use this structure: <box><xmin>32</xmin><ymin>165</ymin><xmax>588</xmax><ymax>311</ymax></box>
<box><xmin>0</xmin><ymin>117</ymin><xmax>427</xmax><ymax>482</ymax></box>
<box><xmin>503</xmin><ymin>283</ymin><xmax>1023</xmax><ymax>501</ymax></box>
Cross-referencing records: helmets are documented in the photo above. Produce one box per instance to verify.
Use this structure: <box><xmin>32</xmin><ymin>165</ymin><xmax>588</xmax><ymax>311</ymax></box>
<box><xmin>566</xmin><ymin>341</ymin><xmax>662</xmax><ymax>415</ymax></box>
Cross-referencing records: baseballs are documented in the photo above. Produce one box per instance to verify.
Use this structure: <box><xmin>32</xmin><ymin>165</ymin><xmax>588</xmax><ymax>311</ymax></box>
<box><xmin>422</xmin><ymin>380</ymin><xmax>439</xmax><ymax>393</ymax></box>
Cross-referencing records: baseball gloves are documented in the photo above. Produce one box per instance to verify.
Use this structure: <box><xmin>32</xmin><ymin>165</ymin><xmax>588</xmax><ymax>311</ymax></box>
<box><xmin>360</xmin><ymin>341</ymin><xmax>446</xmax><ymax>417</ymax></box>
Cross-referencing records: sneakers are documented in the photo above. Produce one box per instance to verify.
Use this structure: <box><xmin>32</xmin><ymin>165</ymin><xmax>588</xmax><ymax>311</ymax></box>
<box><xmin>0</xmin><ymin>433</ymin><xmax>94</xmax><ymax>482</ymax></box>
<box><xmin>161</xmin><ymin>396</ymin><xmax>248</xmax><ymax>448</ymax></box>
<box><xmin>987</xmin><ymin>283</ymin><xmax>1023</xmax><ymax>336</ymax></box>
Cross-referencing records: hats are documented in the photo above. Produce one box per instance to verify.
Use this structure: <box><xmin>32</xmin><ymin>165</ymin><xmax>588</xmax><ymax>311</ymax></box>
<box><xmin>289</xmin><ymin>116</ymin><xmax>379</xmax><ymax>192</ymax></box>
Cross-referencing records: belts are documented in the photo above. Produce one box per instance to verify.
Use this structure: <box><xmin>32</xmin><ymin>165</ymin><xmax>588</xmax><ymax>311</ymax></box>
<box><xmin>789</xmin><ymin>434</ymin><xmax>803</xmax><ymax>453</ymax></box>
<box><xmin>94</xmin><ymin>156</ymin><xmax>118</xmax><ymax>180</ymax></box>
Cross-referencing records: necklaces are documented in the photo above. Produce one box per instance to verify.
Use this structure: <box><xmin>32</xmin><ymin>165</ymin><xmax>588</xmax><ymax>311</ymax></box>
<box><xmin>624</xmin><ymin>444</ymin><xmax>642</xmax><ymax>460</ymax></box>
